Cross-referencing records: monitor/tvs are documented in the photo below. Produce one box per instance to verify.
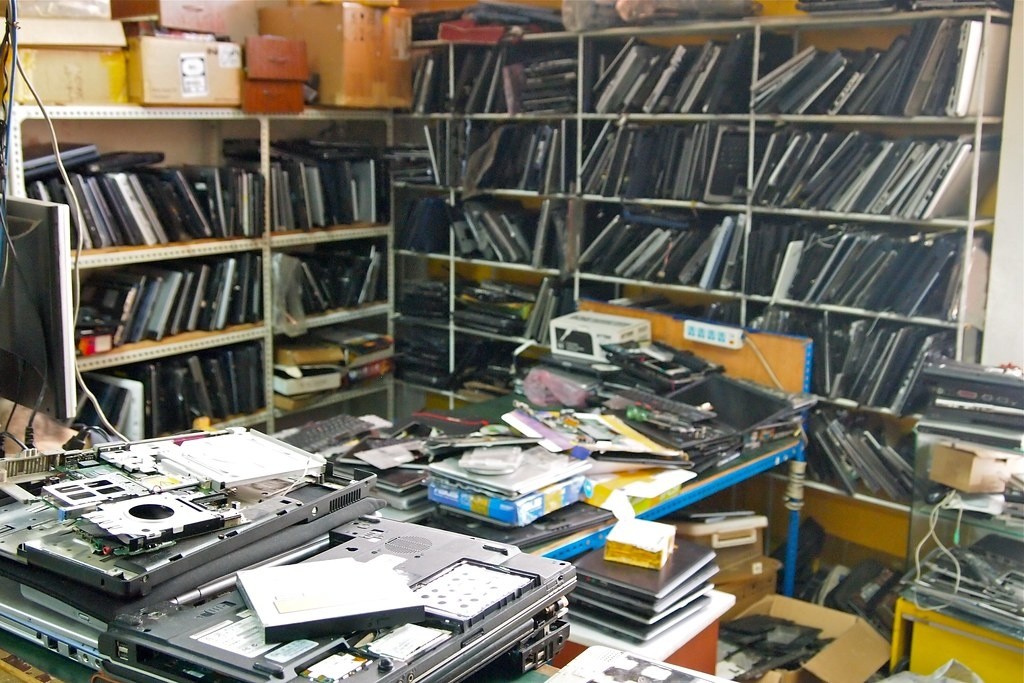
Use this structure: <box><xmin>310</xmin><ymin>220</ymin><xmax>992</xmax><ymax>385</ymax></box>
<box><xmin>0</xmin><ymin>193</ymin><xmax>76</xmax><ymax>421</ymax></box>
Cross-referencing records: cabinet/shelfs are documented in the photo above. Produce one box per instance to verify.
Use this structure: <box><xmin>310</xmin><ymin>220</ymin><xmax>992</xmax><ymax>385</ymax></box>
<box><xmin>1</xmin><ymin>1</ymin><xmax>1023</xmax><ymax>683</ymax></box>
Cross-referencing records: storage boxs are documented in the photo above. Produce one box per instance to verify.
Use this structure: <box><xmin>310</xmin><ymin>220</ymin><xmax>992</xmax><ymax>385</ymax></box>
<box><xmin>666</xmin><ymin>519</ymin><xmax>784</xmax><ymax>623</ymax></box>
<box><xmin>239</xmin><ymin>74</ymin><xmax>306</xmax><ymax>116</ymax></box>
<box><xmin>886</xmin><ymin>420</ymin><xmax>1024</xmax><ymax>683</ymax></box>
<box><xmin>123</xmin><ymin>36</ymin><xmax>244</xmax><ymax>110</ymax></box>
<box><xmin>256</xmin><ymin>2</ymin><xmax>415</xmax><ymax>113</ymax></box>
<box><xmin>109</xmin><ymin>0</ymin><xmax>227</xmax><ymax>40</ymax></box>
<box><xmin>718</xmin><ymin>591</ymin><xmax>894</xmax><ymax>683</ymax></box>
<box><xmin>0</xmin><ymin>16</ymin><xmax>130</xmax><ymax>110</ymax></box>
<box><xmin>240</xmin><ymin>34</ymin><xmax>309</xmax><ymax>81</ymax></box>
